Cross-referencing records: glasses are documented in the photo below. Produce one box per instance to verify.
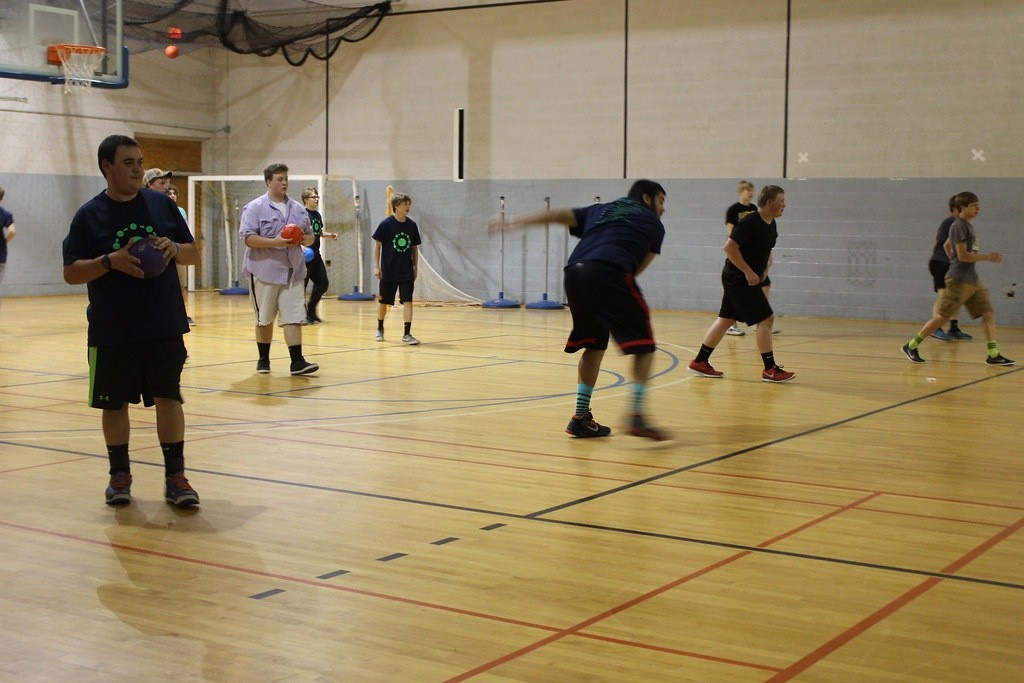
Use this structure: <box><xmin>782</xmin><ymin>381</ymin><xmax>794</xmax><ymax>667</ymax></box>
<box><xmin>308</xmin><ymin>196</ymin><xmax>319</xmax><ymax>199</ymax></box>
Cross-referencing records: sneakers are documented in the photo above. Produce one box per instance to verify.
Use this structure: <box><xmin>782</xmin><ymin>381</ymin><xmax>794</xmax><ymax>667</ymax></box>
<box><xmin>257</xmin><ymin>360</ymin><xmax>270</xmax><ymax>373</ymax></box>
<box><xmin>290</xmin><ymin>361</ymin><xmax>319</xmax><ymax>374</ymax></box>
<box><xmin>375</xmin><ymin>331</ymin><xmax>383</xmax><ymax>341</ymax></box>
<box><xmin>565</xmin><ymin>412</ymin><xmax>611</xmax><ymax>438</ymax></box>
<box><xmin>622</xmin><ymin>418</ymin><xmax>667</xmax><ymax>441</ymax></box>
<box><xmin>947</xmin><ymin>329</ymin><xmax>972</xmax><ymax>340</ymax></box>
<box><xmin>930</xmin><ymin>328</ymin><xmax>949</xmax><ymax>340</ymax></box>
<box><xmin>402</xmin><ymin>335</ymin><xmax>420</xmax><ymax>344</ymax></box>
<box><xmin>165</xmin><ymin>476</ymin><xmax>200</xmax><ymax>504</ymax></box>
<box><xmin>105</xmin><ymin>472</ymin><xmax>132</xmax><ymax>504</ymax></box>
<box><xmin>901</xmin><ymin>343</ymin><xmax>925</xmax><ymax>364</ymax></box>
<box><xmin>985</xmin><ymin>352</ymin><xmax>1016</xmax><ymax>366</ymax></box>
<box><xmin>188</xmin><ymin>317</ymin><xmax>197</xmax><ymax>325</ymax></box>
<box><xmin>725</xmin><ymin>326</ymin><xmax>745</xmax><ymax>336</ymax></box>
<box><xmin>688</xmin><ymin>361</ymin><xmax>724</xmax><ymax>377</ymax></box>
<box><xmin>762</xmin><ymin>366</ymin><xmax>796</xmax><ymax>382</ymax></box>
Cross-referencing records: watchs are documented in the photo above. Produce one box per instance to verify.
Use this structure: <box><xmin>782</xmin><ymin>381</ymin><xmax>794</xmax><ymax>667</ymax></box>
<box><xmin>101</xmin><ymin>254</ymin><xmax>112</xmax><ymax>270</ymax></box>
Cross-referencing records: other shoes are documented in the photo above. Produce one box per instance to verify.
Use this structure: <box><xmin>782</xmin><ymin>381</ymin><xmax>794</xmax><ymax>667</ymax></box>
<box><xmin>306</xmin><ymin>315</ymin><xmax>314</xmax><ymax>325</ymax></box>
<box><xmin>311</xmin><ymin>314</ymin><xmax>322</xmax><ymax>324</ymax></box>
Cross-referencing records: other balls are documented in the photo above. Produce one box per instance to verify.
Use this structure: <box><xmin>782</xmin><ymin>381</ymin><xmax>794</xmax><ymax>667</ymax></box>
<box><xmin>281</xmin><ymin>223</ymin><xmax>303</xmax><ymax>244</ymax></box>
<box><xmin>127</xmin><ymin>238</ymin><xmax>168</xmax><ymax>278</ymax></box>
<box><xmin>304</xmin><ymin>247</ymin><xmax>315</xmax><ymax>264</ymax></box>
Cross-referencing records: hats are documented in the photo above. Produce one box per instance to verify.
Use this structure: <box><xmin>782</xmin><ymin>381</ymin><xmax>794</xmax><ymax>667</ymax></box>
<box><xmin>142</xmin><ymin>168</ymin><xmax>172</xmax><ymax>185</ymax></box>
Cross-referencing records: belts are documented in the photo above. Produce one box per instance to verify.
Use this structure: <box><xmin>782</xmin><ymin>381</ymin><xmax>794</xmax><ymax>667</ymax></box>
<box><xmin>575</xmin><ymin>263</ymin><xmax>589</xmax><ymax>269</ymax></box>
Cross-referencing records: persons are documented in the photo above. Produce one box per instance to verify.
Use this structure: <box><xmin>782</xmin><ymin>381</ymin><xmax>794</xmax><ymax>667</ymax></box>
<box><xmin>62</xmin><ymin>135</ymin><xmax>199</xmax><ymax>506</ymax></box>
<box><xmin>487</xmin><ymin>179</ymin><xmax>673</xmax><ymax>441</ymax></box>
<box><xmin>724</xmin><ymin>180</ymin><xmax>780</xmax><ymax>336</ymax></box>
<box><xmin>372</xmin><ymin>193</ymin><xmax>422</xmax><ymax>345</ymax></box>
<box><xmin>239</xmin><ymin>163</ymin><xmax>319</xmax><ymax>375</ymax></box>
<box><xmin>141</xmin><ymin>168</ymin><xmax>191</xmax><ymax>361</ymax></box>
<box><xmin>0</xmin><ymin>186</ymin><xmax>16</xmax><ymax>284</ymax></box>
<box><xmin>900</xmin><ymin>192</ymin><xmax>1016</xmax><ymax>366</ymax></box>
<box><xmin>928</xmin><ymin>196</ymin><xmax>972</xmax><ymax>341</ymax></box>
<box><xmin>165</xmin><ymin>184</ymin><xmax>198</xmax><ymax>325</ymax></box>
<box><xmin>687</xmin><ymin>185</ymin><xmax>796</xmax><ymax>383</ymax></box>
<box><xmin>300</xmin><ymin>187</ymin><xmax>337</xmax><ymax>325</ymax></box>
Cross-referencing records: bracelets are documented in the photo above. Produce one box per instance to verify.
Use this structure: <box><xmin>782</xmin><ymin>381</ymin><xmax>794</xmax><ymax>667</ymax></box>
<box><xmin>375</xmin><ymin>266</ymin><xmax>379</xmax><ymax>268</ymax></box>
<box><xmin>175</xmin><ymin>243</ymin><xmax>179</xmax><ymax>255</ymax></box>
